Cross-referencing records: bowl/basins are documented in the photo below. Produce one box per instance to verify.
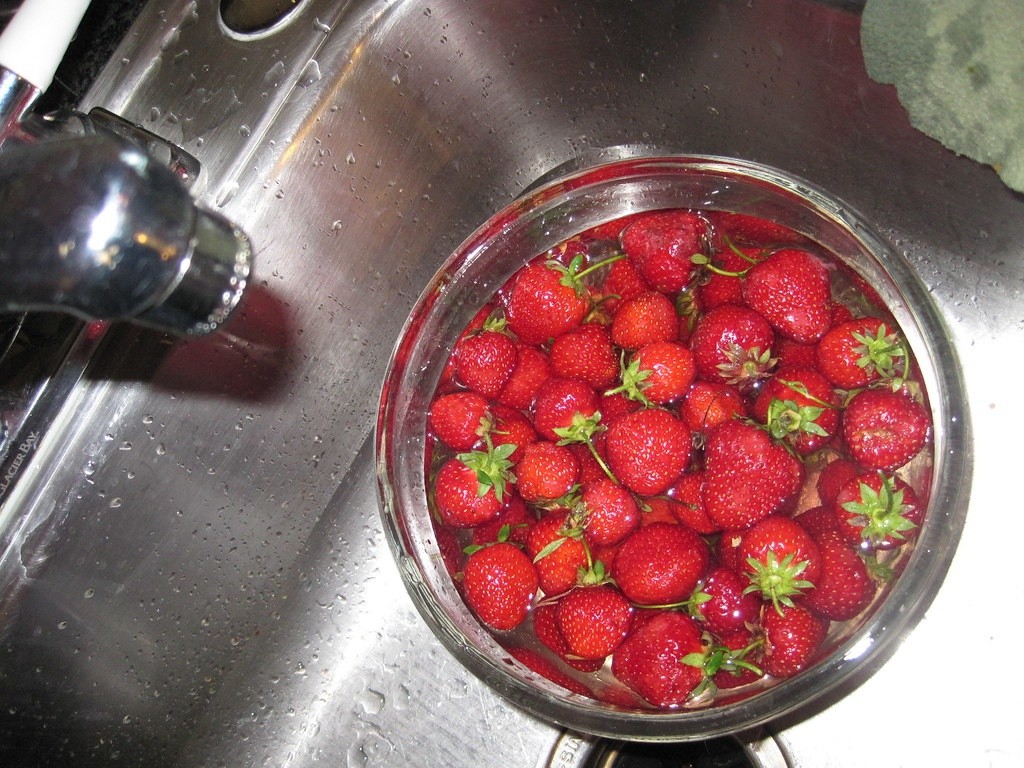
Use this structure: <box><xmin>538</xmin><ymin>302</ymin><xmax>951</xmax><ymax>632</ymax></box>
<box><xmin>371</xmin><ymin>148</ymin><xmax>970</xmax><ymax>743</ymax></box>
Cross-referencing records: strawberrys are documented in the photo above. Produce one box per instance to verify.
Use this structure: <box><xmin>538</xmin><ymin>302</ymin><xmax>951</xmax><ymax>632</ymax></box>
<box><xmin>429</xmin><ymin>210</ymin><xmax>932</xmax><ymax>709</ymax></box>
